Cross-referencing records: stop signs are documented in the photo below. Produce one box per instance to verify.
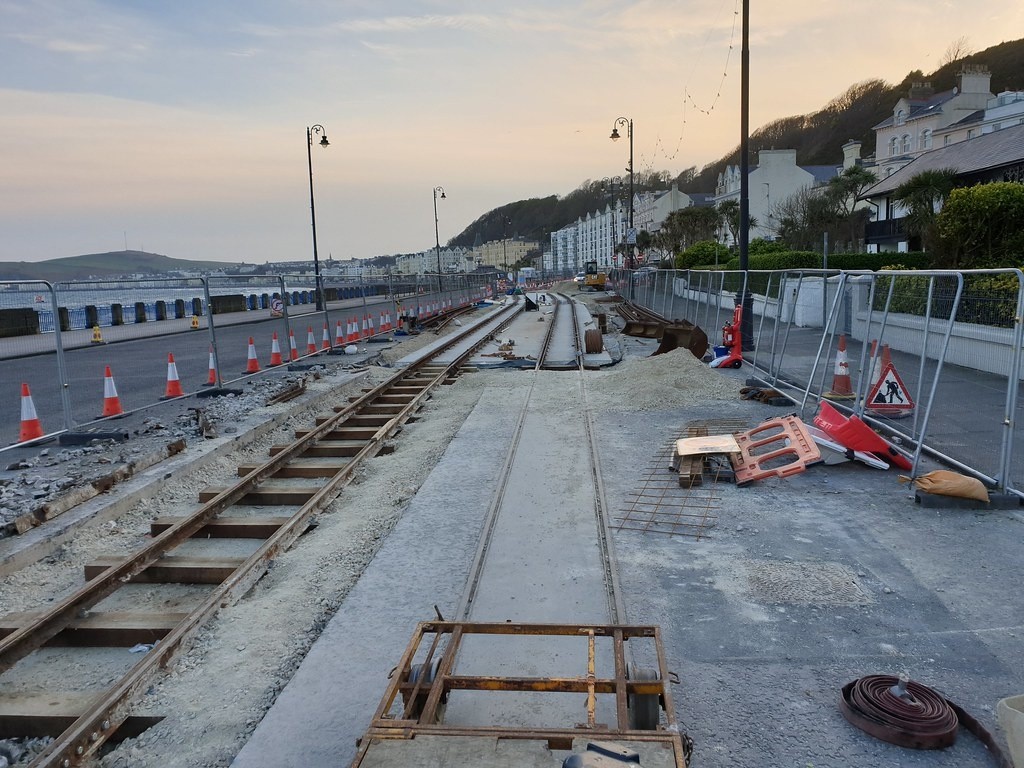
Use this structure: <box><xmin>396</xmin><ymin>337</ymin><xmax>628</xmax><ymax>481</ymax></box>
<box><xmin>637</xmin><ymin>255</ymin><xmax>643</xmax><ymax>261</ymax></box>
<box><xmin>612</xmin><ymin>255</ymin><xmax>617</xmax><ymax>260</ymax></box>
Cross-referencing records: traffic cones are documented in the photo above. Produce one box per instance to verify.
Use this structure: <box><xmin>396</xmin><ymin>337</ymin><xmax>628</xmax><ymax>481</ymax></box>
<box><xmin>242</xmin><ymin>336</ymin><xmax>265</xmax><ymax>374</ymax></box>
<box><xmin>265</xmin><ymin>331</ymin><xmax>287</xmax><ymax>366</ymax></box>
<box><xmin>9</xmin><ymin>383</ymin><xmax>55</xmax><ymax>447</ymax></box>
<box><xmin>158</xmin><ymin>351</ymin><xmax>192</xmax><ymax>400</ymax></box>
<box><xmin>396</xmin><ymin>292</ymin><xmax>485</xmax><ymax>329</ymax></box>
<box><xmin>202</xmin><ymin>345</ymin><xmax>226</xmax><ymax>386</ymax></box>
<box><xmin>95</xmin><ymin>367</ymin><xmax>133</xmax><ymax>420</ymax></box>
<box><xmin>822</xmin><ymin>331</ymin><xmax>912</xmax><ymax>420</ymax></box>
<box><xmin>303</xmin><ymin>311</ymin><xmax>392</xmax><ymax>357</ymax></box>
<box><xmin>283</xmin><ymin>329</ymin><xmax>302</xmax><ymax>362</ymax></box>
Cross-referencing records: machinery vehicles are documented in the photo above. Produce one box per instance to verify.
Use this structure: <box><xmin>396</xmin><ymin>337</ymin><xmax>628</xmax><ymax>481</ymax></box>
<box><xmin>583</xmin><ymin>262</ymin><xmax>606</xmax><ymax>291</ymax></box>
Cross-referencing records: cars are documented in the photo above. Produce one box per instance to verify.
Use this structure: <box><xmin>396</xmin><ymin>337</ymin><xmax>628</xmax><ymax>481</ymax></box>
<box><xmin>628</xmin><ymin>267</ymin><xmax>659</xmax><ymax>285</ymax></box>
<box><xmin>574</xmin><ymin>272</ymin><xmax>585</xmax><ymax>282</ymax></box>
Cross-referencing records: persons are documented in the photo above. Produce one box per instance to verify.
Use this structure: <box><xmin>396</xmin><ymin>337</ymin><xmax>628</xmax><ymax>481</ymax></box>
<box><xmin>589</xmin><ymin>266</ymin><xmax>593</xmax><ymax>271</ymax></box>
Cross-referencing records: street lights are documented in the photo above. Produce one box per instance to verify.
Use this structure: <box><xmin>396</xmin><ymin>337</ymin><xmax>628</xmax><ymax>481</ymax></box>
<box><xmin>600</xmin><ymin>174</ymin><xmax>623</xmax><ymax>269</ymax></box>
<box><xmin>434</xmin><ymin>186</ymin><xmax>447</xmax><ymax>293</ymax></box>
<box><xmin>610</xmin><ymin>116</ymin><xmax>633</xmax><ymax>270</ymax></box>
<box><xmin>503</xmin><ymin>215</ymin><xmax>511</xmax><ymax>273</ymax></box>
<box><xmin>306</xmin><ymin>124</ymin><xmax>330</xmax><ymax>311</ymax></box>
